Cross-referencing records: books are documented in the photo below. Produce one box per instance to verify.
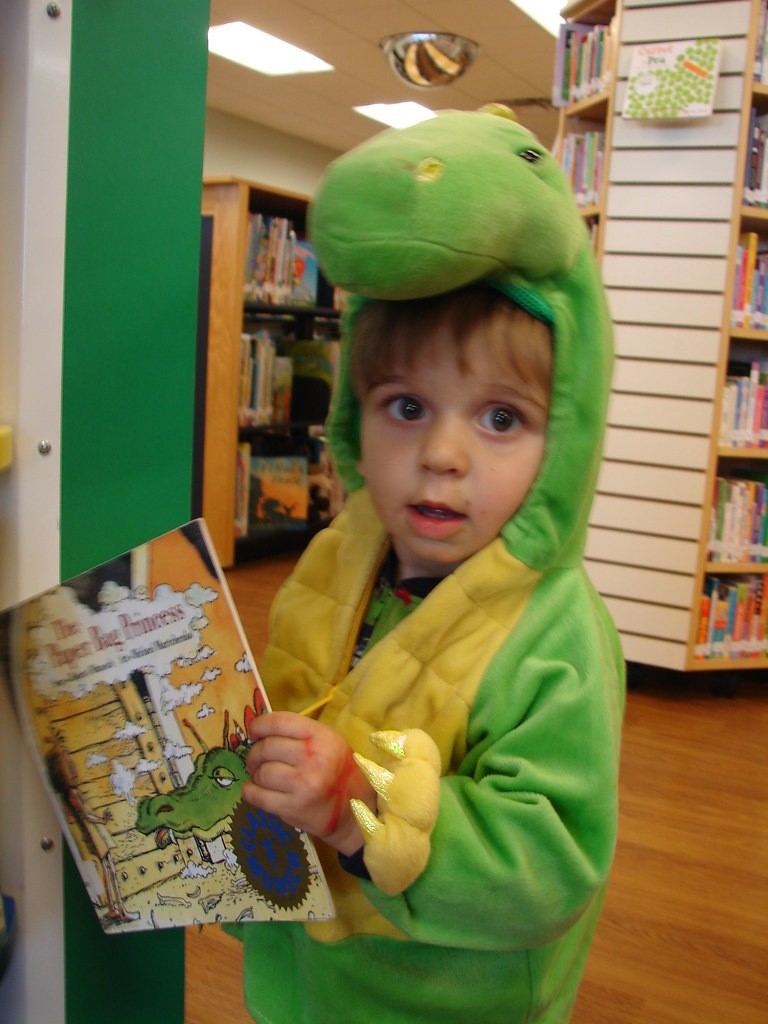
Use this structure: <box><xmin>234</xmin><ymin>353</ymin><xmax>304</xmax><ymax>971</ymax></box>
<box><xmin>223</xmin><ymin>1</ymin><xmax>766</xmax><ymax>675</ymax></box>
<box><xmin>0</xmin><ymin>516</ymin><xmax>335</xmax><ymax>934</ymax></box>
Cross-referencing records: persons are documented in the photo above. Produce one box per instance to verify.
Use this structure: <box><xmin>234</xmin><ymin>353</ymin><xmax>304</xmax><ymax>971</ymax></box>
<box><xmin>154</xmin><ymin>99</ymin><xmax>626</xmax><ymax>1022</ymax></box>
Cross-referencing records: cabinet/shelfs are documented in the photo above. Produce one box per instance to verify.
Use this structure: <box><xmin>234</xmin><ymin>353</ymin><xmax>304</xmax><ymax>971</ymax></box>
<box><xmin>585</xmin><ymin>4</ymin><xmax>768</xmax><ymax>680</ymax></box>
<box><xmin>538</xmin><ymin>1</ymin><xmax>621</xmax><ymax>253</ymax></box>
<box><xmin>236</xmin><ymin>182</ymin><xmax>359</xmax><ymax>568</ymax></box>
